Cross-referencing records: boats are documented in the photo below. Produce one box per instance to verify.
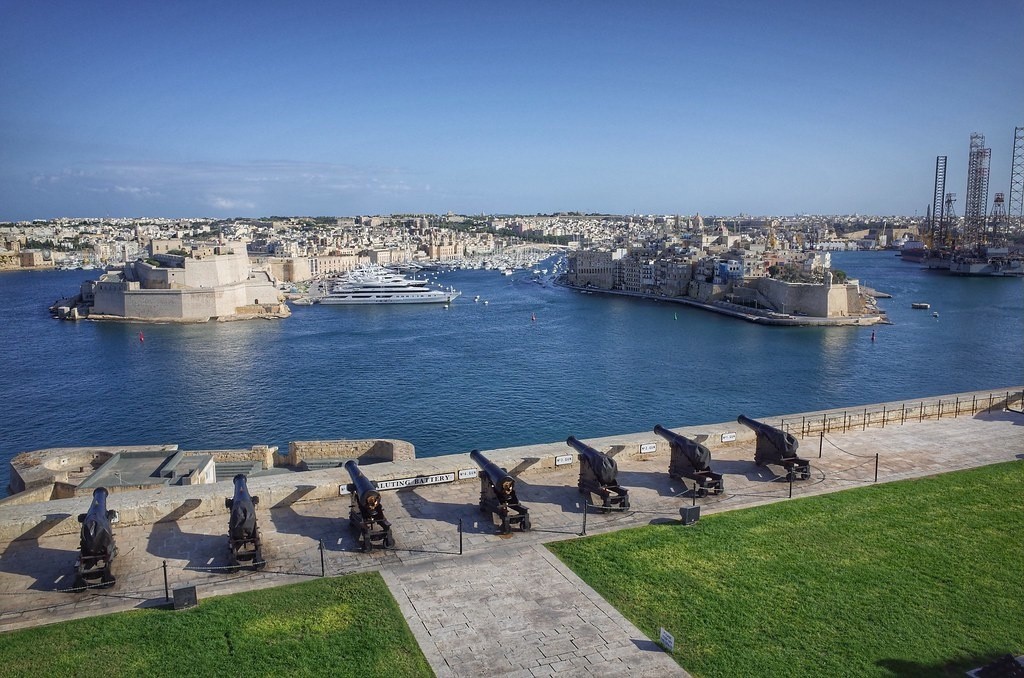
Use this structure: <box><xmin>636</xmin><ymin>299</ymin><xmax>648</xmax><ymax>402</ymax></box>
<box><xmin>898</xmin><ymin>188</ymin><xmax>1024</xmax><ymax>279</ymax></box>
<box><xmin>317</xmin><ymin>261</ymin><xmax>462</xmax><ymax>305</ymax></box>
